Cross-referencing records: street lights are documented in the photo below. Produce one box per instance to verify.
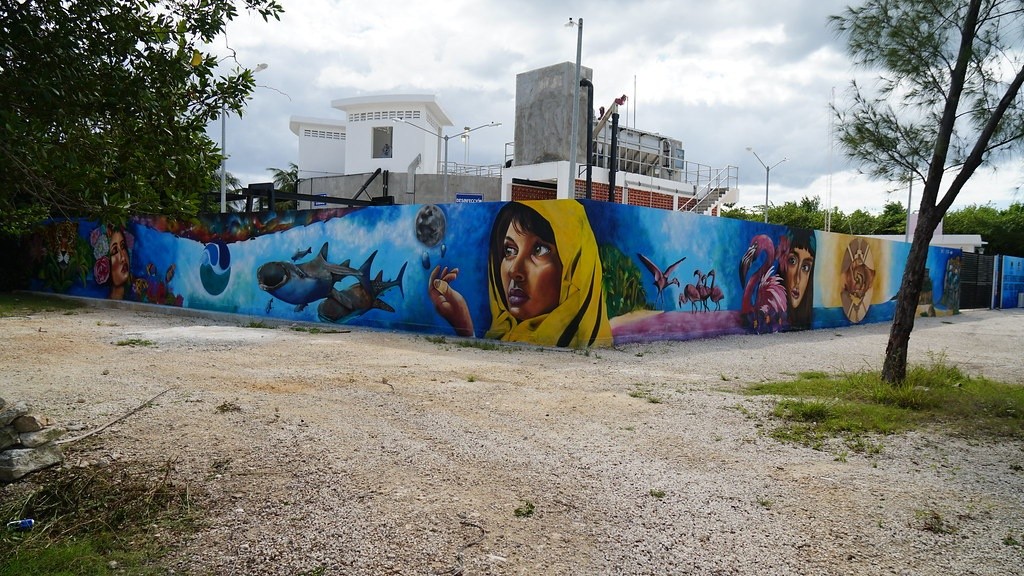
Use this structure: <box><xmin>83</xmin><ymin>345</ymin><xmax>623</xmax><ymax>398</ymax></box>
<box><xmin>221</xmin><ymin>64</ymin><xmax>270</xmax><ymax>213</ymax></box>
<box><xmin>564</xmin><ymin>17</ymin><xmax>584</xmax><ymax>198</ymax></box>
<box><xmin>392</xmin><ymin>117</ymin><xmax>504</xmax><ymax>203</ymax></box>
<box><xmin>746</xmin><ymin>147</ymin><xmax>789</xmax><ymax>223</ymax></box>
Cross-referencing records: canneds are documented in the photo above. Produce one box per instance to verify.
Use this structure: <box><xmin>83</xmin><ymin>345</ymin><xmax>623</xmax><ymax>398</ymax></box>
<box><xmin>7</xmin><ymin>519</ymin><xmax>34</xmax><ymax>532</ymax></box>
